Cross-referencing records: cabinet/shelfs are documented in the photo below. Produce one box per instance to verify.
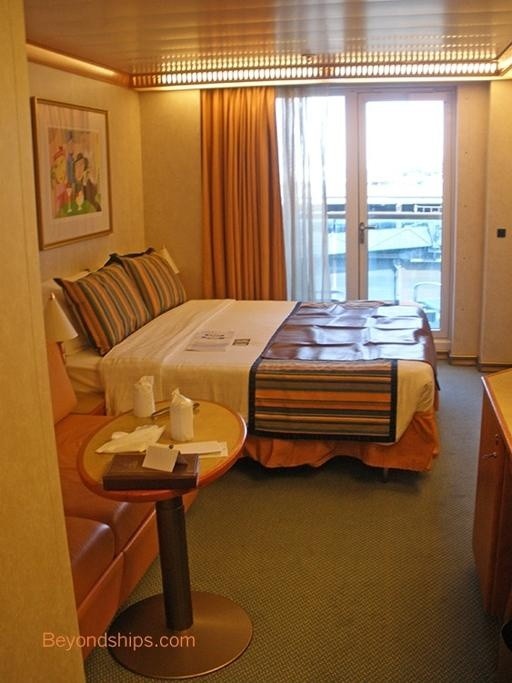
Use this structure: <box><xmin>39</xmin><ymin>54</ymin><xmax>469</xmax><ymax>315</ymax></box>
<box><xmin>467</xmin><ymin>389</ymin><xmax>512</xmax><ymax>618</ymax></box>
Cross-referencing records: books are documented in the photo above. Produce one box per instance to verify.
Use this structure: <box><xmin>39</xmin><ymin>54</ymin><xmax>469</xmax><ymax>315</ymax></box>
<box><xmin>103</xmin><ymin>453</ymin><xmax>199</xmax><ymax>490</ymax></box>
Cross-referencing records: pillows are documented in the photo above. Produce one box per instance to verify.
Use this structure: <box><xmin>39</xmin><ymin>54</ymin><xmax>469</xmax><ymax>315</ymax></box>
<box><xmin>53</xmin><ymin>243</ymin><xmax>186</xmax><ymax>357</ymax></box>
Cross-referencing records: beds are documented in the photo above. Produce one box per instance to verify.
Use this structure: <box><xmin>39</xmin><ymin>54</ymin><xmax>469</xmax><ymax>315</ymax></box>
<box><xmin>40</xmin><ymin>269</ymin><xmax>439</xmax><ymax>479</ymax></box>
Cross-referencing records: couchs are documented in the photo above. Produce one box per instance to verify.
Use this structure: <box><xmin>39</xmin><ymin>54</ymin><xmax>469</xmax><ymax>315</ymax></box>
<box><xmin>43</xmin><ymin>320</ymin><xmax>207</xmax><ymax>658</ymax></box>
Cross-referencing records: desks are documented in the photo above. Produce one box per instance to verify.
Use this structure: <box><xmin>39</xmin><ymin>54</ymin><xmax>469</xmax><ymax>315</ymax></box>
<box><xmin>76</xmin><ymin>394</ymin><xmax>258</xmax><ymax>679</ymax></box>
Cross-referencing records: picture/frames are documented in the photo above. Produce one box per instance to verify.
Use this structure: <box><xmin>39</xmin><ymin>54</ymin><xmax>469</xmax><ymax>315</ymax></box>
<box><xmin>30</xmin><ymin>96</ymin><xmax>117</xmax><ymax>253</ymax></box>
